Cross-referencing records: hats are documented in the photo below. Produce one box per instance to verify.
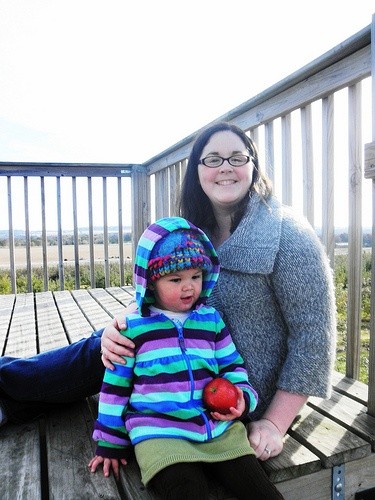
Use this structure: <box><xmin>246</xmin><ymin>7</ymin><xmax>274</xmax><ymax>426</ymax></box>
<box><xmin>147</xmin><ymin>228</ymin><xmax>213</xmax><ymax>285</ymax></box>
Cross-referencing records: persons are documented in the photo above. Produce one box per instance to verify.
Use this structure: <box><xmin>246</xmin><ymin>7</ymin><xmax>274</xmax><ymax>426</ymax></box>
<box><xmin>0</xmin><ymin>123</ymin><xmax>336</xmax><ymax>460</ymax></box>
<box><xmin>88</xmin><ymin>216</ymin><xmax>285</xmax><ymax>500</ymax></box>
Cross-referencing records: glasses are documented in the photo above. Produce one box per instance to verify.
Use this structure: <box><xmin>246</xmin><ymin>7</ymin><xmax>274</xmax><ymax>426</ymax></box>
<box><xmin>195</xmin><ymin>154</ymin><xmax>253</xmax><ymax>168</ymax></box>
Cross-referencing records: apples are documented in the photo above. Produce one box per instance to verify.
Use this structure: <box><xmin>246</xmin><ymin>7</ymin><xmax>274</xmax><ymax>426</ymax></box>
<box><xmin>204</xmin><ymin>378</ymin><xmax>239</xmax><ymax>415</ymax></box>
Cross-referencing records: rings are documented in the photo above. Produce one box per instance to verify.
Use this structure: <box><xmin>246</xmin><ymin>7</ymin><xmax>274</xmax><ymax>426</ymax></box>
<box><xmin>265</xmin><ymin>448</ymin><xmax>271</xmax><ymax>454</ymax></box>
